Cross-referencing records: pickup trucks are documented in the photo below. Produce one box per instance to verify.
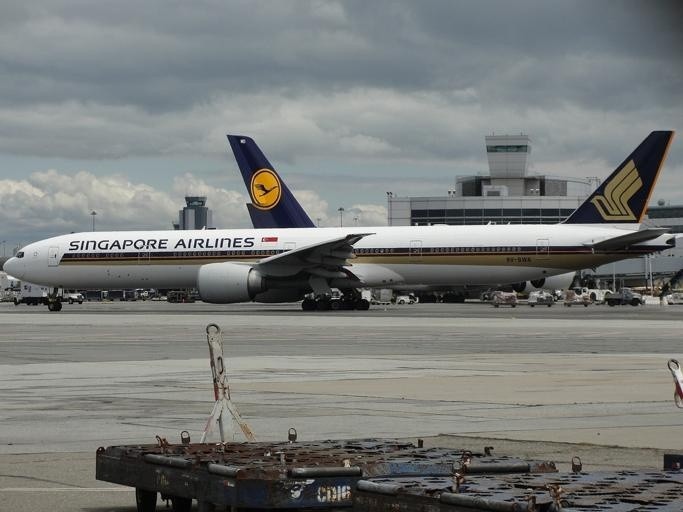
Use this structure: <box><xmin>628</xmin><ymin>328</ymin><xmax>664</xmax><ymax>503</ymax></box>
<box><xmin>602</xmin><ymin>287</ymin><xmax>643</xmax><ymax>307</ymax></box>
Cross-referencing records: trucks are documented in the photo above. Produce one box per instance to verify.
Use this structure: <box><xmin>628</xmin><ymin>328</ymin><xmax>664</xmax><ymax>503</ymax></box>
<box><xmin>20</xmin><ymin>281</ymin><xmax>85</xmax><ymax>306</ymax></box>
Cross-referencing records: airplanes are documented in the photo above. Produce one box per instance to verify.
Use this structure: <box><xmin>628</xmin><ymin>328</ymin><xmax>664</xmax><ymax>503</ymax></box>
<box><xmin>2</xmin><ymin>131</ymin><xmax>682</xmax><ymax>315</ymax></box>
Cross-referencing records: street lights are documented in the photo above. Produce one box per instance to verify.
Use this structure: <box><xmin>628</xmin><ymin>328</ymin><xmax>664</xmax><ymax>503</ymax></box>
<box><xmin>337</xmin><ymin>207</ymin><xmax>345</xmax><ymax>227</ymax></box>
<box><xmin>89</xmin><ymin>210</ymin><xmax>97</xmax><ymax>232</ymax></box>
<box><xmin>316</xmin><ymin>217</ymin><xmax>322</xmax><ymax>227</ymax></box>
